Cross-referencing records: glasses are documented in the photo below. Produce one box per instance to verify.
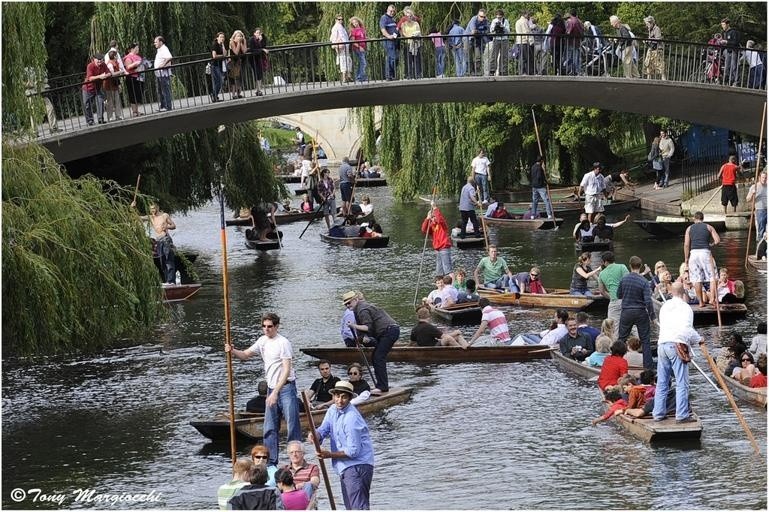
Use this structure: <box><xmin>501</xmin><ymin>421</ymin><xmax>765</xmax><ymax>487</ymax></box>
<box><xmin>531</xmin><ymin>274</ymin><xmax>538</xmax><ymax>276</ymax></box>
<box><xmin>345</xmin><ymin>302</ymin><xmax>350</xmax><ymax>306</ymax></box>
<box><xmin>349</xmin><ymin>372</ymin><xmax>358</xmax><ymax>375</ymax></box>
<box><xmin>741</xmin><ymin>359</ymin><xmax>750</xmax><ymax>362</ymax></box>
<box><xmin>263</xmin><ymin>325</ymin><xmax>273</xmax><ymax>328</ymax></box>
<box><xmin>255</xmin><ymin>456</ymin><xmax>268</xmax><ymax>459</ymax></box>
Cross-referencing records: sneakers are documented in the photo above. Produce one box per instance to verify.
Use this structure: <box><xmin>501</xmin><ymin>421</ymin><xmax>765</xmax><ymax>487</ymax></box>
<box><xmin>210</xmin><ymin>91</ymin><xmax>262</xmax><ymax>103</ymax></box>
<box><xmin>548</xmin><ymin>215</ymin><xmax>556</xmax><ymax>219</ymax></box>
<box><xmin>663</xmin><ymin>416</ymin><xmax>668</xmax><ymax>420</ymax></box>
<box><xmin>370</xmin><ymin>388</ymin><xmax>389</xmax><ymax>395</ymax></box>
<box><xmin>482</xmin><ymin>200</ymin><xmax>488</xmax><ymax>204</ymax></box>
<box><xmin>654</xmin><ymin>182</ymin><xmax>667</xmax><ymax>190</ymax></box>
<box><xmin>88</xmin><ymin>112</ymin><xmax>144</xmax><ymax>125</ymax></box>
<box><xmin>155</xmin><ymin>107</ymin><xmax>168</xmax><ymax>111</ymax></box>
<box><xmin>531</xmin><ymin>216</ymin><xmax>535</xmax><ymax>219</ymax></box>
<box><xmin>676</xmin><ymin>417</ymin><xmax>697</xmax><ymax>423</ymax></box>
<box><xmin>50</xmin><ymin>128</ymin><xmax>63</xmax><ymax>133</ymax></box>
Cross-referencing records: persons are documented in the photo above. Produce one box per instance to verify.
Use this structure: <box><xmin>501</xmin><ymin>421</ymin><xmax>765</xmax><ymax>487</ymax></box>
<box><xmin>249</xmin><ymin>28</ymin><xmax>270</xmax><ymax>99</ymax></box>
<box><xmin>103</xmin><ymin>52</ymin><xmax>126</xmax><ymax>119</ymax></box>
<box><xmin>82</xmin><ymin>54</ymin><xmax>112</xmax><ymax>125</ymax></box>
<box><xmin>706</xmin><ymin>18</ymin><xmax>767</xmax><ymax>91</ymax></box>
<box><xmin>330</xmin><ymin>5</ymin><xmax>670</xmax><ymax>83</ymax></box>
<box><xmin>151</xmin><ymin>33</ymin><xmax>175</xmax><ymax>114</ymax></box>
<box><xmin>227</xmin><ymin>29</ymin><xmax>251</xmax><ymax>98</ymax></box>
<box><xmin>104</xmin><ymin>41</ymin><xmax>126</xmax><ymax>109</ymax></box>
<box><xmin>19</xmin><ymin>55</ymin><xmax>64</xmax><ymax>138</ymax></box>
<box><xmin>218</xmin><ymin>126</ymin><xmax>767</xmax><ymax>509</ymax></box>
<box><xmin>126</xmin><ymin>40</ymin><xmax>145</xmax><ymax>117</ymax></box>
<box><xmin>129</xmin><ymin>200</ymin><xmax>181</xmax><ymax>286</ymax></box>
<box><xmin>210</xmin><ymin>31</ymin><xmax>229</xmax><ymax>103</ymax></box>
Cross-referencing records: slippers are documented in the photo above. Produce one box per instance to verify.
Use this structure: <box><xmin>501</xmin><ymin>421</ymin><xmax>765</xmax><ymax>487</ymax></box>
<box><xmin>707</xmin><ymin>306</ymin><xmax>717</xmax><ymax>308</ymax></box>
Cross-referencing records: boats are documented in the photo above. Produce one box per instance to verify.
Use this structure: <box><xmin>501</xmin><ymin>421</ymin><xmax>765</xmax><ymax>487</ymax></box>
<box><xmin>746</xmin><ymin>252</ymin><xmax>767</xmax><ymax>270</ymax></box>
<box><xmin>150</xmin><ymin>251</ymin><xmax>198</xmax><ymax>272</ymax></box>
<box><xmin>298</xmin><ymin>342</ymin><xmax>553</xmax><ymax>365</ymax></box>
<box><xmin>101</xmin><ymin>284</ymin><xmax>204</xmax><ymax>303</ymax></box>
<box><xmin>652</xmin><ymin>294</ymin><xmax>747</xmax><ymax>327</ymax></box>
<box><xmin>189</xmin><ymin>386</ymin><xmax>416</xmax><ymax>447</ymax></box>
<box><xmin>631</xmin><ymin>215</ymin><xmax>726</xmax><ymax>238</ymax></box>
<box><xmin>551</xmin><ymin>347</ymin><xmax>660</xmax><ymax>378</ymax></box>
<box><xmin>422</xmin><ymin>180</ymin><xmax>643</xmax><ymax>252</ymax></box>
<box><xmin>221</xmin><ymin>148</ymin><xmax>390</xmax><ymax>248</ymax></box>
<box><xmin>712</xmin><ymin>353</ymin><xmax>767</xmax><ymax>408</ymax></box>
<box><xmin>469</xmin><ymin>285</ymin><xmax>594</xmax><ymax>311</ymax></box>
<box><xmin>598</xmin><ymin>380</ymin><xmax>705</xmax><ymax>448</ymax></box>
<box><xmin>421</xmin><ymin>294</ymin><xmax>484</xmax><ymax>326</ymax></box>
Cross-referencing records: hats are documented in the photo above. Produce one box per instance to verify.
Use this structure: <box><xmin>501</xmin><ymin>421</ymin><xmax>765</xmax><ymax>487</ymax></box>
<box><xmin>343</xmin><ymin>291</ymin><xmax>358</xmax><ymax>305</ymax></box>
<box><xmin>328</xmin><ymin>381</ymin><xmax>358</xmax><ymax>398</ymax></box>
<box><xmin>93</xmin><ymin>54</ymin><xmax>103</xmax><ymax>60</ymax></box>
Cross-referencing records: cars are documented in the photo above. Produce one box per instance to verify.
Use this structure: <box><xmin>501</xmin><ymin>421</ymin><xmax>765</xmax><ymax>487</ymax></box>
<box><xmin>737</xmin><ymin>141</ymin><xmax>767</xmax><ymax>168</ymax></box>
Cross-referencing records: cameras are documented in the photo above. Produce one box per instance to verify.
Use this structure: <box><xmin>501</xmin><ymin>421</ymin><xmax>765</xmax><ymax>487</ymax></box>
<box><xmin>495</xmin><ymin>20</ymin><xmax>501</xmax><ymax>30</ymax></box>
<box><xmin>575</xmin><ymin>345</ymin><xmax>583</xmax><ymax>352</ymax></box>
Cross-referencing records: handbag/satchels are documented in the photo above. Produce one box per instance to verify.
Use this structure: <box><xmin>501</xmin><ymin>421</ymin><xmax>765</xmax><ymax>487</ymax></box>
<box><xmin>648</xmin><ymin>152</ymin><xmax>652</xmax><ymax>161</ymax></box>
<box><xmin>111</xmin><ymin>77</ymin><xmax>121</xmax><ymax>85</ymax></box>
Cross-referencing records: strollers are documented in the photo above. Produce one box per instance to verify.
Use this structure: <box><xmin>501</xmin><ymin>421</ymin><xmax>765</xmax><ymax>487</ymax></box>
<box><xmin>570</xmin><ymin>24</ymin><xmax>623</xmax><ymax>76</ymax></box>
<box><xmin>689</xmin><ymin>33</ymin><xmax>727</xmax><ymax>85</ymax></box>
